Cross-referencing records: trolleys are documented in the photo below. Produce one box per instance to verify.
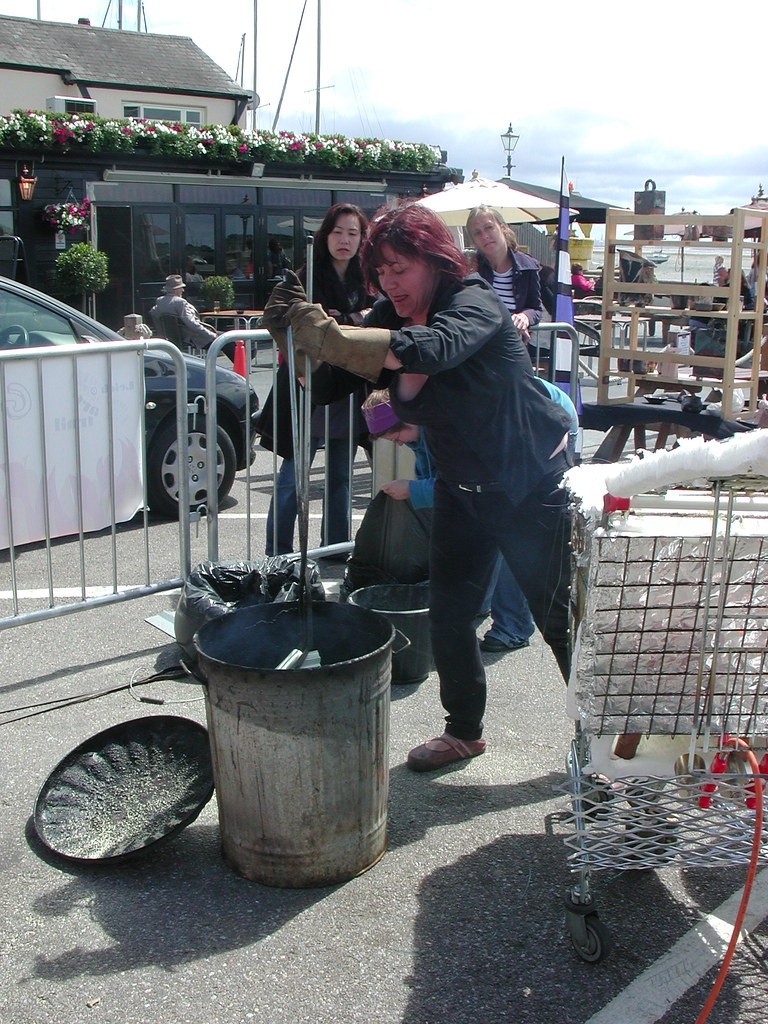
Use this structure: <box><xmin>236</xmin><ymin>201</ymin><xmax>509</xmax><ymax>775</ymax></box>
<box><xmin>562</xmin><ymin>424</ymin><xmax>768</xmax><ymax>966</ymax></box>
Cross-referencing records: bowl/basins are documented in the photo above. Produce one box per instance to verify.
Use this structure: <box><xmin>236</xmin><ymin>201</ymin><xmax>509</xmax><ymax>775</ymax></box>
<box><xmin>695</xmin><ymin>303</ymin><xmax>725</xmax><ymax>310</ymax></box>
<box><xmin>643</xmin><ymin>394</ymin><xmax>669</xmax><ymax>404</ymax></box>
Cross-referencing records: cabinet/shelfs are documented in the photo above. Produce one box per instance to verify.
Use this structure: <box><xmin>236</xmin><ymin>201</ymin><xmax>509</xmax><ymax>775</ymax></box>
<box><xmin>598</xmin><ymin>208</ymin><xmax>768</xmax><ymax>422</ymax></box>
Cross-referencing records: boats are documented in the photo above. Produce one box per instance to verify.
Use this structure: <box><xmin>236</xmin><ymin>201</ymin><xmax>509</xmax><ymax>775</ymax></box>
<box><xmin>646</xmin><ymin>253</ymin><xmax>670</xmax><ymax>264</ymax></box>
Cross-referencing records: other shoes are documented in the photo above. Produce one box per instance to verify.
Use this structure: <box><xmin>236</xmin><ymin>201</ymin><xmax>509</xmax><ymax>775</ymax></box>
<box><xmin>318</xmin><ymin>546</ymin><xmax>352</xmax><ymax>564</ymax></box>
<box><xmin>408</xmin><ymin>732</ymin><xmax>485</xmax><ymax>771</ymax></box>
<box><xmin>481</xmin><ymin>636</ymin><xmax>509</xmax><ymax>651</ymax></box>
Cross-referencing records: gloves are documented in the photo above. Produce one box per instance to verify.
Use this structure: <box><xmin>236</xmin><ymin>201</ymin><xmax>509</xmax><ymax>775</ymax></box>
<box><xmin>282</xmin><ymin>302</ymin><xmax>391</xmax><ymax>384</ymax></box>
<box><xmin>261</xmin><ymin>268</ymin><xmax>323</xmax><ymax>378</ymax></box>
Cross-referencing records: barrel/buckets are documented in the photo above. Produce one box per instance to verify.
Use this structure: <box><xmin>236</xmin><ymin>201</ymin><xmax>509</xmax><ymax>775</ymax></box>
<box><xmin>193</xmin><ymin>599</ymin><xmax>411</xmax><ymax>889</ymax></box>
<box><xmin>346</xmin><ymin>583</ymin><xmax>433</xmax><ymax>685</ymax></box>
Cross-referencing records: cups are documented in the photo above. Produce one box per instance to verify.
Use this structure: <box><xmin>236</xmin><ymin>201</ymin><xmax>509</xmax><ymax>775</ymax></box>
<box><xmin>236</xmin><ymin>303</ymin><xmax>245</xmax><ymax>314</ymax></box>
<box><xmin>740</xmin><ymin>295</ymin><xmax>744</xmax><ymax>311</ymax></box>
<box><xmin>214</xmin><ymin>301</ymin><xmax>221</xmax><ymax>314</ymax></box>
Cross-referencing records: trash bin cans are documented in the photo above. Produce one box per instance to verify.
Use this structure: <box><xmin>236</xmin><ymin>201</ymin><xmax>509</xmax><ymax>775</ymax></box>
<box><xmin>179</xmin><ymin>599</ymin><xmax>411</xmax><ymax>887</ymax></box>
<box><xmin>347</xmin><ymin>583</ymin><xmax>433</xmax><ymax>685</ymax></box>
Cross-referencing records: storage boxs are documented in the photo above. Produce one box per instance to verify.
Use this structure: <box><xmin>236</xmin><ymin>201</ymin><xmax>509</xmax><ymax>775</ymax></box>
<box><xmin>569</xmin><ymin>237</ymin><xmax>594</xmax><ymax>270</ymax></box>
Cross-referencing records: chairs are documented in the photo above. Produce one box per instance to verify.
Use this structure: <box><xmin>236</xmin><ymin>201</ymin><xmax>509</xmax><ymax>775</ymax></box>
<box><xmin>573</xmin><ymin>318</ymin><xmax>613</xmax><ymax>357</ymax></box>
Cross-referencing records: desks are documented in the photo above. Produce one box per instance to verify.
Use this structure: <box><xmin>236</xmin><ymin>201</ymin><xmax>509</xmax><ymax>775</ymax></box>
<box><xmin>574</xmin><ymin>315</ymin><xmax>650</xmax><ymax>353</ymax></box>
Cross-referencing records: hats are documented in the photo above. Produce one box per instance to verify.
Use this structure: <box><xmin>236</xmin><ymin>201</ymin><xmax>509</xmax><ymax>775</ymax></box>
<box><xmin>162</xmin><ymin>275</ymin><xmax>186</xmax><ymax>289</ymax></box>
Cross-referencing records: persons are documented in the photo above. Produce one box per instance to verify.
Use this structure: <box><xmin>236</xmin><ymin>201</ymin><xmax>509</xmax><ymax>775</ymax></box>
<box><xmin>186</xmin><ymin>237</ymin><xmax>293</xmax><ymax>287</ymax></box>
<box><xmin>571</xmin><ymin>263</ymin><xmax>619</xmax><ymax>301</ymax></box>
<box><xmin>361</xmin><ymin>376</ymin><xmax>579</xmax><ymax>652</ymax></box>
<box><xmin>465</xmin><ymin>206</ymin><xmax>542</xmax><ymax>346</ymax></box>
<box><xmin>259</xmin><ymin>202</ymin><xmax>383</xmax><ymax>562</ymax></box>
<box><xmin>150</xmin><ymin>275</ymin><xmax>257</xmax><ymax>364</ymax></box>
<box><xmin>262</xmin><ymin>205</ymin><xmax>642</xmax><ymax>774</ymax></box>
<box><xmin>713</xmin><ymin>254</ymin><xmax>768</xmax><ymax>339</ymax></box>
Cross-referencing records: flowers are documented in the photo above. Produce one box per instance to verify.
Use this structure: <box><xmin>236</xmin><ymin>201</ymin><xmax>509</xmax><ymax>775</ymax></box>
<box><xmin>46</xmin><ymin>198</ymin><xmax>89</xmax><ymax>235</ymax></box>
<box><xmin>0</xmin><ymin>107</ymin><xmax>442</xmax><ymax>173</ymax></box>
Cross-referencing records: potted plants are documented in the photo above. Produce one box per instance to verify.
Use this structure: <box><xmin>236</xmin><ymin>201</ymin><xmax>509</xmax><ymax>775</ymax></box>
<box><xmin>200</xmin><ymin>274</ymin><xmax>234</xmax><ymax>329</ymax></box>
<box><xmin>55</xmin><ymin>243</ymin><xmax>110</xmax><ymax>296</ymax></box>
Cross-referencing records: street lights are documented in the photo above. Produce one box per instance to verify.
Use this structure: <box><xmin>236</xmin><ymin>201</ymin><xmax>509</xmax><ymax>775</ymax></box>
<box><xmin>501</xmin><ymin>122</ymin><xmax>520</xmax><ymax>179</ymax></box>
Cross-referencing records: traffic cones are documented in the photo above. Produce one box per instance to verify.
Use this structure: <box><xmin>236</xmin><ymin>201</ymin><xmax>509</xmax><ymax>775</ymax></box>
<box><xmin>232</xmin><ymin>339</ymin><xmax>247</xmax><ymax>377</ymax></box>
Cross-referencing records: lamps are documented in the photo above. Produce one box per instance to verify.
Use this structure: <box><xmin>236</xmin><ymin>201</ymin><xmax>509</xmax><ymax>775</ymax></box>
<box><xmin>16</xmin><ymin>165</ymin><xmax>36</xmax><ymax>202</ymax></box>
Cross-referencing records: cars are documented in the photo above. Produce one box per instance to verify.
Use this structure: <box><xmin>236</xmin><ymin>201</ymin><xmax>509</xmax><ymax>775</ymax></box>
<box><xmin>0</xmin><ymin>277</ymin><xmax>259</xmax><ymax>521</ymax></box>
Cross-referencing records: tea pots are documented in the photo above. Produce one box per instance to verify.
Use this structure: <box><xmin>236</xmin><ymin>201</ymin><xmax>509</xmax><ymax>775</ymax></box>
<box><xmin>681</xmin><ymin>396</ymin><xmax>704</xmax><ymax>414</ymax></box>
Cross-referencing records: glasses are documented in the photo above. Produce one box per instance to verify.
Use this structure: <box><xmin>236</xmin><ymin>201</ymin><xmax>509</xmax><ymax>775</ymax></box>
<box><xmin>392</xmin><ymin>431</ymin><xmax>400</xmax><ymax>444</ymax></box>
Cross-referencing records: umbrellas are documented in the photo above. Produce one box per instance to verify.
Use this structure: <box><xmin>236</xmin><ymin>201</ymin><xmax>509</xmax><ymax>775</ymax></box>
<box><xmin>409</xmin><ymin>169</ymin><xmax>579</xmax><ymax>226</ymax></box>
<box><xmin>494</xmin><ymin>177</ymin><xmax>626</xmax><ymax>224</ymax></box>
